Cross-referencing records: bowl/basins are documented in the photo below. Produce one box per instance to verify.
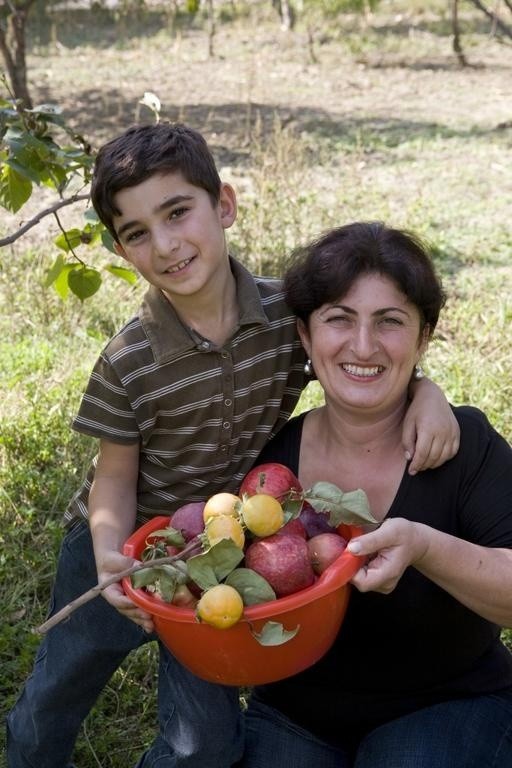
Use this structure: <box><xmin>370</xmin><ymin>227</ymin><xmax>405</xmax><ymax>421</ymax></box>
<box><xmin>120</xmin><ymin>500</ymin><xmax>369</xmax><ymax>688</ymax></box>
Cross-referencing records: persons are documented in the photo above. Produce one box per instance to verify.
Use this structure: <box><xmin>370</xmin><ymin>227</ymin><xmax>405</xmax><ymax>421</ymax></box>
<box><xmin>225</xmin><ymin>214</ymin><xmax>511</xmax><ymax>765</ymax></box>
<box><xmin>1</xmin><ymin>115</ymin><xmax>461</xmax><ymax>764</ymax></box>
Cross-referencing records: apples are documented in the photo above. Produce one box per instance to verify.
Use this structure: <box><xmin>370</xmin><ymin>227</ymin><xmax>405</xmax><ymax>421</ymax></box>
<box><xmin>154</xmin><ymin>464</ymin><xmax>347</xmax><ymax>605</ymax></box>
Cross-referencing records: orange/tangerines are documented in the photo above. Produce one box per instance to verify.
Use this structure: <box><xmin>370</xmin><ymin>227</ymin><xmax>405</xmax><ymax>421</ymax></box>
<box><xmin>196</xmin><ymin>586</ymin><xmax>243</xmax><ymax>631</ymax></box>
